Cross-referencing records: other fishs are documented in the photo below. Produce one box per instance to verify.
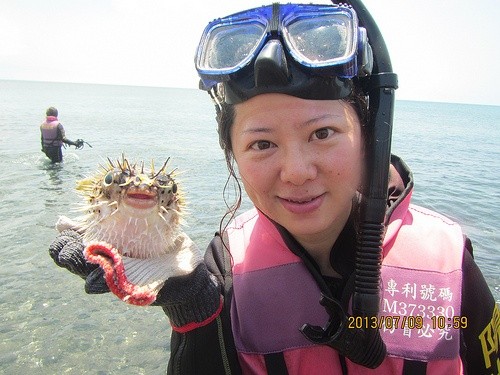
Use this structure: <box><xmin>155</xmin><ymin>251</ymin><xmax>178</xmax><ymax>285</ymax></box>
<box><xmin>54</xmin><ymin>151</ymin><xmax>190</xmax><ymax>261</ymax></box>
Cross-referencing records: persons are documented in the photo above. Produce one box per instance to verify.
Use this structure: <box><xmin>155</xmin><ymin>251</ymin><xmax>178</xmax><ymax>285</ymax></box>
<box><xmin>48</xmin><ymin>0</ymin><xmax>500</xmax><ymax>375</ymax></box>
<box><xmin>40</xmin><ymin>106</ymin><xmax>81</xmax><ymax>163</ymax></box>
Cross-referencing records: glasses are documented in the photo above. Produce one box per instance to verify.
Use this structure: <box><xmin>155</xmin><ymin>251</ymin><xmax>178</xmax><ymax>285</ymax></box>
<box><xmin>194</xmin><ymin>2</ymin><xmax>372</xmax><ymax>106</ymax></box>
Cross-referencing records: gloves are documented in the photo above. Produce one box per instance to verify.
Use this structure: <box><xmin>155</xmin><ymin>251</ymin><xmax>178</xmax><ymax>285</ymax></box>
<box><xmin>58</xmin><ymin>203</ymin><xmax>206</xmax><ymax>308</ymax></box>
<box><xmin>49</xmin><ymin>229</ymin><xmax>222</xmax><ymax>332</ymax></box>
